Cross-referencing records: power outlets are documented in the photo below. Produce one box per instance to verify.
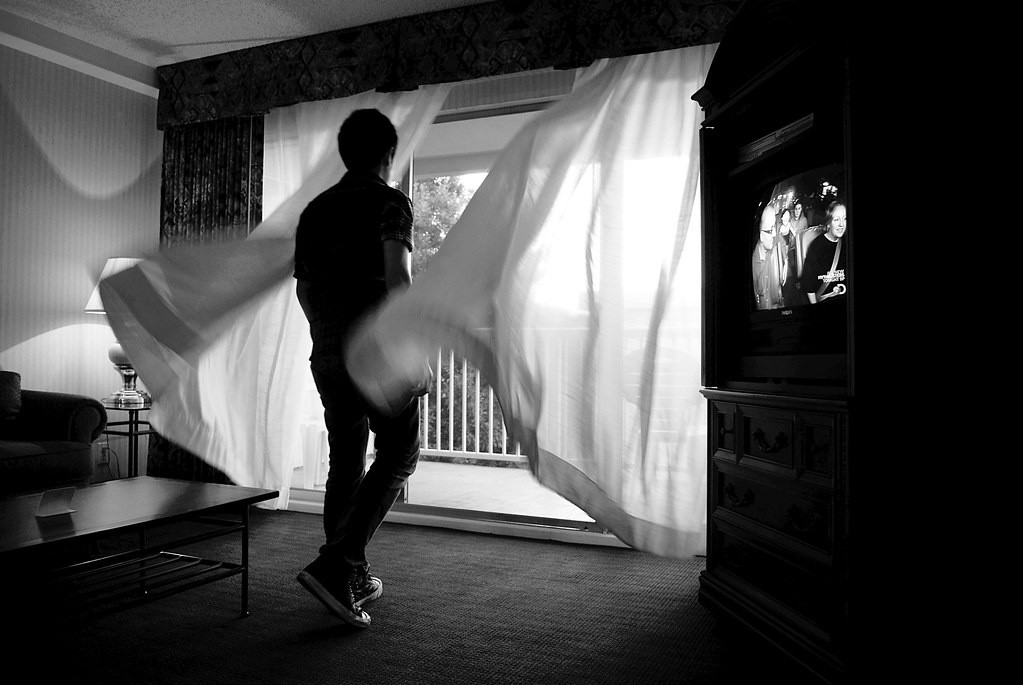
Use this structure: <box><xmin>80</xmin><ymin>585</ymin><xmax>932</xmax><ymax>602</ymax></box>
<box><xmin>97</xmin><ymin>443</ymin><xmax>109</xmax><ymax>464</ymax></box>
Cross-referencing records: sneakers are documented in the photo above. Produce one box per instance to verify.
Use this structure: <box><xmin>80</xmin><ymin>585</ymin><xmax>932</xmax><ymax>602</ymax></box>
<box><xmin>298</xmin><ymin>554</ymin><xmax>371</xmax><ymax>629</ymax></box>
<box><xmin>349</xmin><ymin>560</ymin><xmax>382</xmax><ymax>606</ymax></box>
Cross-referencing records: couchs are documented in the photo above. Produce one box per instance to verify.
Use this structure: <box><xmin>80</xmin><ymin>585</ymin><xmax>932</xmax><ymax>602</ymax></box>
<box><xmin>0</xmin><ymin>369</ymin><xmax>110</xmax><ymax>500</ymax></box>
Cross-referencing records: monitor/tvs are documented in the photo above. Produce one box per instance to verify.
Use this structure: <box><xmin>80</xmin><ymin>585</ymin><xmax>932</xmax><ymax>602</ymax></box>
<box><xmin>745</xmin><ymin>151</ymin><xmax>849</xmax><ymax>355</ymax></box>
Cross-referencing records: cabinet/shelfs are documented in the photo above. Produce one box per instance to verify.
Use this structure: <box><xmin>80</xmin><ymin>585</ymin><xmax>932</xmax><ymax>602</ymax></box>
<box><xmin>697</xmin><ymin>379</ymin><xmax>849</xmax><ymax>685</ymax></box>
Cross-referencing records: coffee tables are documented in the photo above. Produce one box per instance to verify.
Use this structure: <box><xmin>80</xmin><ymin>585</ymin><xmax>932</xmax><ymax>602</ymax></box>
<box><xmin>0</xmin><ymin>474</ymin><xmax>280</xmax><ymax>656</ymax></box>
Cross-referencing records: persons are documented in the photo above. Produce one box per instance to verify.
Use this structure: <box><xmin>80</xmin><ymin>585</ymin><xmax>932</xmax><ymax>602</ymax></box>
<box><xmin>752</xmin><ymin>199</ymin><xmax>847</xmax><ymax>310</ymax></box>
<box><xmin>294</xmin><ymin>108</ymin><xmax>434</xmax><ymax>630</ymax></box>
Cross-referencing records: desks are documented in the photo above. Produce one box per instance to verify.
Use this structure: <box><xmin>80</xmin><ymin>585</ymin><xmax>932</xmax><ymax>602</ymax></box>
<box><xmin>100</xmin><ymin>399</ymin><xmax>161</xmax><ymax>479</ymax></box>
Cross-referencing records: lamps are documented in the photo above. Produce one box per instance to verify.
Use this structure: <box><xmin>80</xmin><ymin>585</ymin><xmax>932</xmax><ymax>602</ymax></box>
<box><xmin>83</xmin><ymin>258</ymin><xmax>154</xmax><ymax>404</ymax></box>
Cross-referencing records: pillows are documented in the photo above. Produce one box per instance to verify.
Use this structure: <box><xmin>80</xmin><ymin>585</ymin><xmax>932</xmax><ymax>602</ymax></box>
<box><xmin>0</xmin><ymin>368</ymin><xmax>21</xmax><ymax>443</ymax></box>
